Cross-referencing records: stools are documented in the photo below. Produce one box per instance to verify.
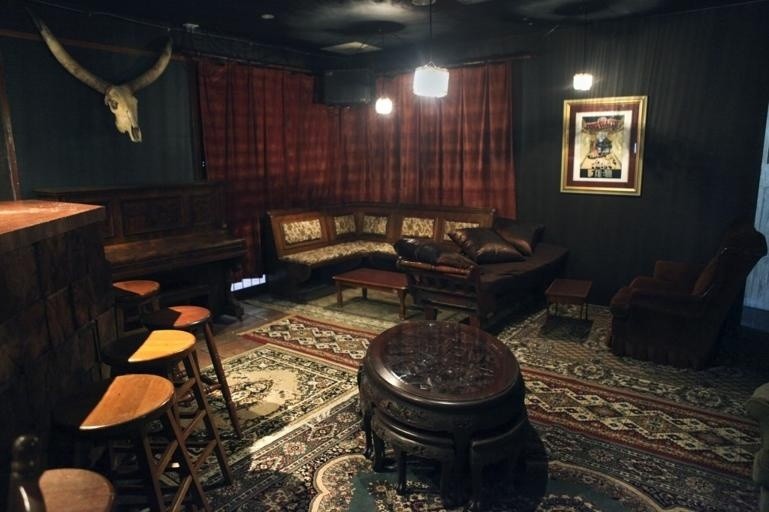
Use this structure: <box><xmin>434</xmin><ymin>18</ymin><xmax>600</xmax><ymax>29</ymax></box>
<box><xmin>14</xmin><ymin>465</ymin><xmax>117</xmax><ymax>512</ymax></box>
<box><xmin>102</xmin><ymin>328</ymin><xmax>234</xmax><ymax>487</ymax></box>
<box><xmin>111</xmin><ymin>279</ymin><xmax>164</xmax><ymax>337</ymax></box>
<box><xmin>48</xmin><ymin>372</ymin><xmax>211</xmax><ymax>512</ymax></box>
<box><xmin>141</xmin><ymin>302</ymin><xmax>246</xmax><ymax>440</ymax></box>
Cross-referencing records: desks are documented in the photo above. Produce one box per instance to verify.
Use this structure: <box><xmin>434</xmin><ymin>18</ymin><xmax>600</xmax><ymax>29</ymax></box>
<box><xmin>357</xmin><ymin>320</ymin><xmax>529</xmax><ymax>509</ymax></box>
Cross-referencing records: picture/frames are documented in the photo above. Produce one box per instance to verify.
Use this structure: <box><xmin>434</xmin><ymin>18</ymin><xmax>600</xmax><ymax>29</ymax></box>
<box><xmin>558</xmin><ymin>94</ymin><xmax>650</xmax><ymax>198</ymax></box>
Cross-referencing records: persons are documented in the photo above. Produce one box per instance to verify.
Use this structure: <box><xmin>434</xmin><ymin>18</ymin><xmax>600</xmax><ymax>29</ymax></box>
<box><xmin>586</xmin><ymin>116</ymin><xmax>618</xmax><ymax>156</ymax></box>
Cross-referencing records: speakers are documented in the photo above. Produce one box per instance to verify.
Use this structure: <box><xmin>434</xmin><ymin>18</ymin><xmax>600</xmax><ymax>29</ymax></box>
<box><xmin>322</xmin><ymin>69</ymin><xmax>375</xmax><ymax>104</ymax></box>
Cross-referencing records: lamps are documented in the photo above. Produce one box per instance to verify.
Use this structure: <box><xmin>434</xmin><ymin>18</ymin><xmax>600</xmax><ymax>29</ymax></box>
<box><xmin>569</xmin><ymin>1</ymin><xmax>597</xmax><ymax>93</ymax></box>
<box><xmin>407</xmin><ymin>0</ymin><xmax>451</xmax><ymax>98</ymax></box>
<box><xmin>370</xmin><ymin>26</ymin><xmax>396</xmax><ymax>116</ymax></box>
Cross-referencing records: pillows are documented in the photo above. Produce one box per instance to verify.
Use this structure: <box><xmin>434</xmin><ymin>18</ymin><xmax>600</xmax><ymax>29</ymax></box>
<box><xmin>444</xmin><ymin>226</ymin><xmax>526</xmax><ymax>268</ymax></box>
<box><xmin>393</xmin><ymin>236</ymin><xmax>482</xmax><ymax>276</ymax></box>
<box><xmin>493</xmin><ymin>215</ymin><xmax>548</xmax><ymax>257</ymax></box>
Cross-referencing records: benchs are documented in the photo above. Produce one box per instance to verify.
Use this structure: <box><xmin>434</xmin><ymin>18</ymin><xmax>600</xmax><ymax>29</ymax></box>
<box><xmin>395</xmin><ymin>215</ymin><xmax>571</xmax><ymax>332</ymax></box>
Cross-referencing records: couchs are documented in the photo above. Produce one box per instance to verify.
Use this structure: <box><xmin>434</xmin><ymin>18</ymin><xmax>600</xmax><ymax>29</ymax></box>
<box><xmin>259</xmin><ymin>200</ymin><xmax>498</xmax><ymax>304</ymax></box>
<box><xmin>604</xmin><ymin>221</ymin><xmax>769</xmax><ymax>373</ymax></box>
